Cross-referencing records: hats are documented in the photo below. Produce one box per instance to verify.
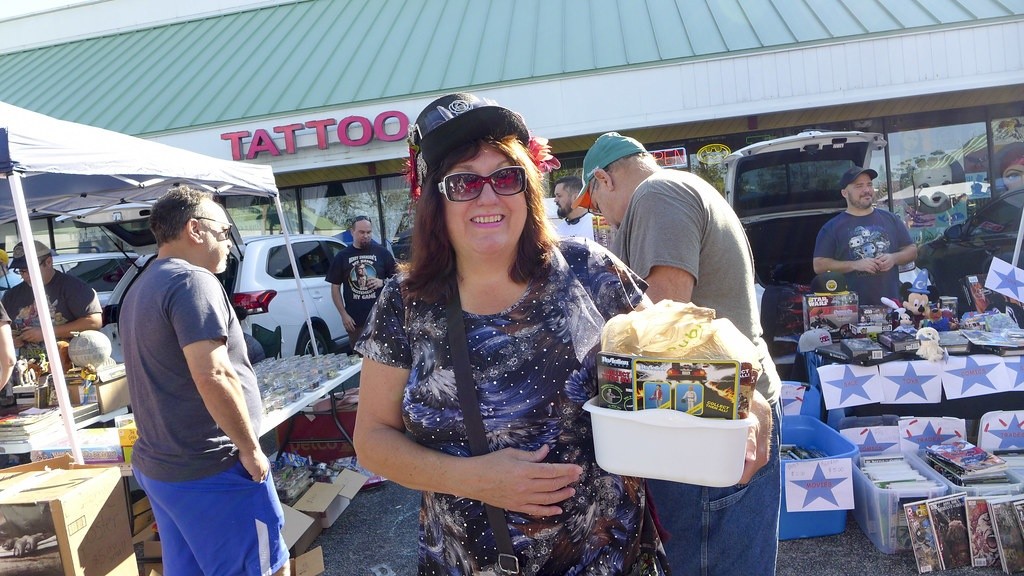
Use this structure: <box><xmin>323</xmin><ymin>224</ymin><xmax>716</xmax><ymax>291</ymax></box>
<box><xmin>841</xmin><ymin>165</ymin><xmax>877</xmax><ymax>190</ymax></box>
<box><xmin>570</xmin><ymin>132</ymin><xmax>647</xmax><ymax>210</ymax></box>
<box><xmin>799</xmin><ymin>326</ymin><xmax>832</xmax><ymax>353</ymax></box>
<box><xmin>811</xmin><ymin>271</ymin><xmax>849</xmax><ymax>295</ymax></box>
<box><xmin>412</xmin><ymin>92</ymin><xmax>529</xmax><ymax>171</ymax></box>
<box><xmin>8</xmin><ymin>240</ymin><xmax>52</xmax><ymax>270</ymax></box>
<box><xmin>0</xmin><ymin>249</ymin><xmax>9</xmax><ymax>265</ymax></box>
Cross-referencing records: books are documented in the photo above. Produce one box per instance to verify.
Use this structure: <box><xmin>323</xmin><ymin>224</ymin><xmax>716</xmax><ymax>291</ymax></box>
<box><xmin>927</xmin><ymin>437</ymin><xmax>1009</xmax><ymax>487</ymax></box>
<box><xmin>0</xmin><ymin>413</ymin><xmax>62</xmax><ymax>444</ymax></box>
<box><xmin>903</xmin><ymin>491</ymin><xmax>1024</xmax><ymax>574</ymax></box>
<box><xmin>860</xmin><ymin>454</ymin><xmax>937</xmax><ymax>547</ymax></box>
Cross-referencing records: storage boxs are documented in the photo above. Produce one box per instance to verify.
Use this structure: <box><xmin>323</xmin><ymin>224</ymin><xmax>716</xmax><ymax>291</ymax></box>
<box><xmin>582</xmin><ymin>395</ymin><xmax>750</xmax><ymax>487</ymax></box>
<box><xmin>775</xmin><ymin>415</ymin><xmax>1007</xmax><ymax>555</ymax></box>
<box><xmin>67</xmin><ymin>377</ymin><xmax>372</xmax><ymax>576</ymax></box>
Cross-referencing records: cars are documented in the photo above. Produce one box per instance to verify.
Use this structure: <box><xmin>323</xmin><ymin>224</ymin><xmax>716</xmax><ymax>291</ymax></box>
<box><xmin>389</xmin><ymin>227</ymin><xmax>416</xmax><ymax>260</ymax></box>
<box><xmin>898</xmin><ymin>188</ymin><xmax>1024</xmax><ymax>298</ymax></box>
<box><xmin>723</xmin><ymin>130</ymin><xmax>889</xmax><ymax>372</ymax></box>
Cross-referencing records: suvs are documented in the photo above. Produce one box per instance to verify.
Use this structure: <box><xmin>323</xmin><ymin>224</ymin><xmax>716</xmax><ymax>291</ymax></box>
<box><xmin>54</xmin><ymin>197</ymin><xmax>354</xmax><ymax>360</ymax></box>
<box><xmin>0</xmin><ymin>248</ymin><xmax>139</xmax><ymax>359</ymax></box>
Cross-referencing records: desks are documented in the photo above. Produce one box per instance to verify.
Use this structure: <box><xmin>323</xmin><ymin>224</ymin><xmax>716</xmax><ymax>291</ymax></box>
<box><xmin>804</xmin><ymin>350</ymin><xmax>1024</xmax><ymax>427</ymax></box>
<box><xmin>249</xmin><ymin>357</ymin><xmax>365</xmax><ymax>469</ymax></box>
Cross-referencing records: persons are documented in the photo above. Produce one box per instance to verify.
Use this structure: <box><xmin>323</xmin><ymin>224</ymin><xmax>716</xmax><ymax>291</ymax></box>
<box><xmin>0</xmin><ymin>300</ymin><xmax>17</xmax><ymax>392</ymax></box>
<box><xmin>325</xmin><ymin>216</ymin><xmax>397</xmax><ymax>354</ymax></box>
<box><xmin>571</xmin><ymin>132</ymin><xmax>782</xmax><ymax>576</ymax></box>
<box><xmin>1</xmin><ymin>240</ymin><xmax>103</xmax><ymax>361</ymax></box>
<box><xmin>813</xmin><ymin>166</ymin><xmax>917</xmax><ymax>305</ymax></box>
<box><xmin>352</xmin><ymin>93</ymin><xmax>772</xmax><ymax>576</ymax></box>
<box><xmin>119</xmin><ymin>186</ymin><xmax>290</xmax><ymax>576</ymax></box>
<box><xmin>554</xmin><ymin>176</ymin><xmax>595</xmax><ymax>239</ymax></box>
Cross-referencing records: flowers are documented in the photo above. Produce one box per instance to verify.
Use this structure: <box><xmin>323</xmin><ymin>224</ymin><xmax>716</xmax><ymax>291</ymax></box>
<box><xmin>527</xmin><ymin>134</ymin><xmax>562</xmax><ymax>173</ymax></box>
<box><xmin>399</xmin><ymin>126</ymin><xmax>425</xmax><ymax>200</ymax></box>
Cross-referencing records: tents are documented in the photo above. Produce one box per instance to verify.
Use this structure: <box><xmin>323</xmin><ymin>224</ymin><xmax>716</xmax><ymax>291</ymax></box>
<box><xmin>0</xmin><ymin>100</ymin><xmax>318</xmax><ymax>459</ymax></box>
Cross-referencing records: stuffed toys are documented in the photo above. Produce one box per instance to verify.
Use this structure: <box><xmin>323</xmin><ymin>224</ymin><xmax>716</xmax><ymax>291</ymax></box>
<box><xmin>881</xmin><ymin>268</ymin><xmax>956</xmax><ymax>361</ymax></box>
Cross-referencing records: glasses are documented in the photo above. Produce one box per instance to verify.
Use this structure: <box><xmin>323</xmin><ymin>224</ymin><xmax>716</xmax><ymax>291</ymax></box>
<box><xmin>13</xmin><ymin>261</ymin><xmax>43</xmax><ymax>275</ymax></box>
<box><xmin>565</xmin><ymin>211</ymin><xmax>589</xmax><ymax>226</ymax></box>
<box><xmin>185</xmin><ymin>215</ymin><xmax>231</xmax><ymax>239</ymax></box>
<box><xmin>435</xmin><ymin>163</ymin><xmax>528</xmax><ymax>201</ymax></box>
<box><xmin>352</xmin><ymin>216</ymin><xmax>371</xmax><ymax>224</ymax></box>
<box><xmin>588</xmin><ymin>166</ymin><xmax>609</xmax><ymax>216</ymax></box>
